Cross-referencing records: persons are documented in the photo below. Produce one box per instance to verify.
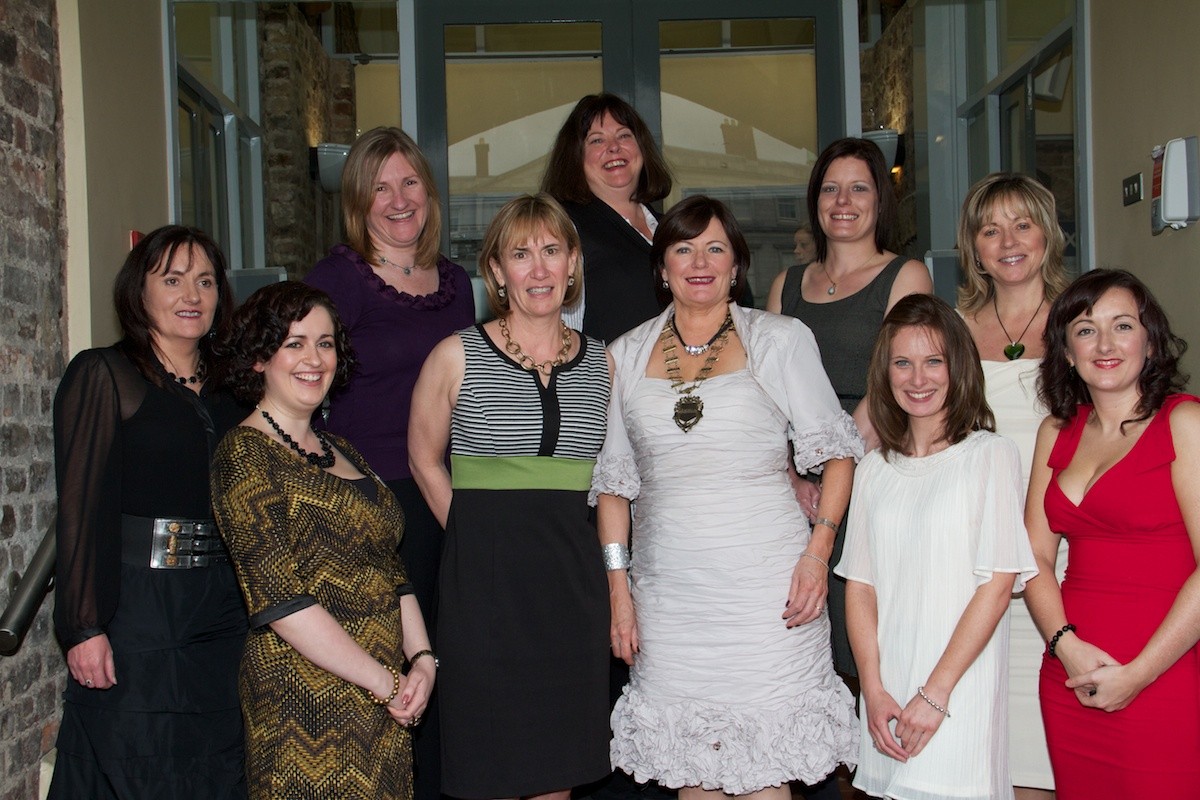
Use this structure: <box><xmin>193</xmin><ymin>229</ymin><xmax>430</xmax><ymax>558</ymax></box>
<box><xmin>44</xmin><ymin>94</ymin><xmax>1200</xmax><ymax>800</ymax></box>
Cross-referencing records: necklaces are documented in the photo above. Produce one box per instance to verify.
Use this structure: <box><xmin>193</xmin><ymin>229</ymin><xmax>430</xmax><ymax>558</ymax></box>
<box><xmin>660</xmin><ymin>313</ymin><xmax>734</xmax><ymax>433</ymax></box>
<box><xmin>374</xmin><ymin>252</ymin><xmax>416</xmax><ymax>275</ymax></box>
<box><xmin>823</xmin><ymin>249</ymin><xmax>878</xmax><ymax>295</ymax></box>
<box><xmin>168</xmin><ymin>361</ymin><xmax>205</xmax><ymax>385</ymax></box>
<box><xmin>994</xmin><ymin>294</ymin><xmax>1044</xmax><ymax>360</ymax></box>
<box><xmin>667</xmin><ymin>317</ymin><xmax>729</xmax><ymax>336</ymax></box>
<box><xmin>499</xmin><ymin>316</ymin><xmax>572</xmax><ymax>375</ymax></box>
<box><xmin>673</xmin><ymin>315</ymin><xmax>733</xmax><ymax>355</ymax></box>
<box><xmin>256</xmin><ymin>405</ymin><xmax>336</xmax><ymax>468</ymax></box>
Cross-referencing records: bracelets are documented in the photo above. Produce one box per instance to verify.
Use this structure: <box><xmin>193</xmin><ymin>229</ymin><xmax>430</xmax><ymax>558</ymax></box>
<box><xmin>816</xmin><ymin>517</ymin><xmax>838</xmax><ymax>532</ymax></box>
<box><xmin>410</xmin><ymin>650</ymin><xmax>439</xmax><ymax>669</ymax></box>
<box><xmin>918</xmin><ymin>687</ymin><xmax>951</xmax><ymax>717</ymax></box>
<box><xmin>1047</xmin><ymin>624</ymin><xmax>1077</xmax><ymax>657</ymax></box>
<box><xmin>802</xmin><ymin>554</ymin><xmax>829</xmax><ymax>569</ymax></box>
<box><xmin>368</xmin><ymin>665</ymin><xmax>400</xmax><ymax>705</ymax></box>
<box><xmin>601</xmin><ymin>543</ymin><xmax>631</xmax><ymax>571</ymax></box>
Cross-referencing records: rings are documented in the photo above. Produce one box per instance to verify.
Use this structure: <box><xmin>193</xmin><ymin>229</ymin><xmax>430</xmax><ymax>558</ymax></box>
<box><xmin>815</xmin><ymin>605</ymin><xmax>822</xmax><ymax>610</ymax></box>
<box><xmin>86</xmin><ymin>679</ymin><xmax>93</xmax><ymax>684</ymax></box>
<box><xmin>414</xmin><ymin>716</ymin><xmax>422</xmax><ymax>727</ymax></box>
<box><xmin>1089</xmin><ymin>690</ymin><xmax>1096</xmax><ymax>695</ymax></box>
